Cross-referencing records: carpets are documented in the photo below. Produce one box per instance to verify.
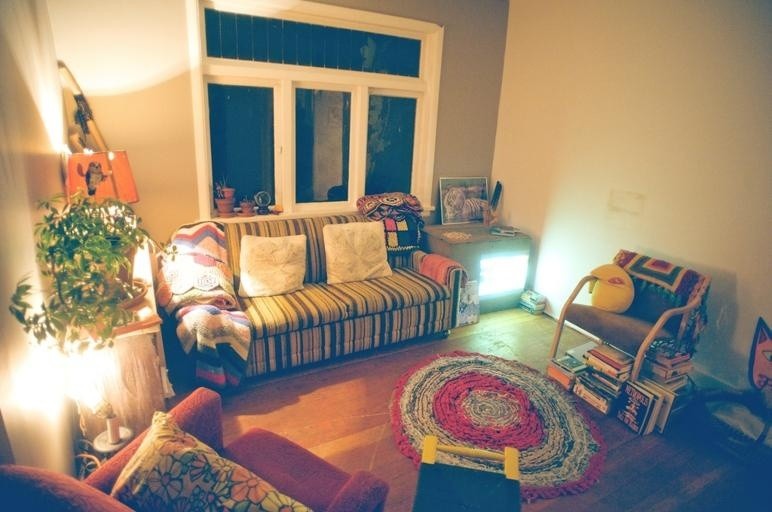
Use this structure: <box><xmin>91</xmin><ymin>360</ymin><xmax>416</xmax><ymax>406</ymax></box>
<box><xmin>390</xmin><ymin>350</ymin><xmax>610</xmax><ymax>503</ymax></box>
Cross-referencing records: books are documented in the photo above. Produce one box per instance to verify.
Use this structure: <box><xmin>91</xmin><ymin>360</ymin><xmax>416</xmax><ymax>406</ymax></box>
<box><xmin>493</xmin><ymin>225</ymin><xmax>521</xmax><ymax>234</ymax></box>
<box><xmin>545</xmin><ymin>335</ymin><xmax>696</xmax><ymax>438</ymax></box>
<box><xmin>518</xmin><ymin>290</ymin><xmax>547</xmax><ymax>315</ymax></box>
<box><xmin>490</xmin><ymin>230</ymin><xmax>516</xmax><ymax>237</ymax></box>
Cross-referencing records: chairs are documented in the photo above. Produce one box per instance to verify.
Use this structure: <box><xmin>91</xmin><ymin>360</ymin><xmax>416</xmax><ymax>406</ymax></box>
<box><xmin>550</xmin><ymin>248</ymin><xmax>713</xmax><ymax>415</ymax></box>
<box><xmin>408</xmin><ymin>435</ymin><xmax>522</xmax><ymax>512</ymax></box>
<box><xmin>0</xmin><ymin>385</ymin><xmax>390</xmax><ymax>512</ymax></box>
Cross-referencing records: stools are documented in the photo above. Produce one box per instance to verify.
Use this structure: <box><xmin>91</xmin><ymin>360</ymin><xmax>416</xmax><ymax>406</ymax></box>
<box><xmin>90</xmin><ymin>427</ymin><xmax>135</xmax><ymax>458</ymax></box>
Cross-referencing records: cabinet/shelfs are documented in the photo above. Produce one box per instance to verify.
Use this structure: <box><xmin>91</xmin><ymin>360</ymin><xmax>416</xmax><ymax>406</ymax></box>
<box><xmin>419</xmin><ymin>222</ymin><xmax>533</xmax><ymax>314</ymax></box>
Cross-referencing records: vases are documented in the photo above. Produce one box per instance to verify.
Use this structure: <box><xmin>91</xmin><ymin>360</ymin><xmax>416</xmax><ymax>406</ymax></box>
<box><xmin>104</xmin><ymin>416</ymin><xmax>121</xmax><ymax>447</ymax></box>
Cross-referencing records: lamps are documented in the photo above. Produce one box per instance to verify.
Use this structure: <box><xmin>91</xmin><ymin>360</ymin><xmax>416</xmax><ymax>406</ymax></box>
<box><xmin>66</xmin><ymin>148</ymin><xmax>140</xmax><ymax>207</ymax></box>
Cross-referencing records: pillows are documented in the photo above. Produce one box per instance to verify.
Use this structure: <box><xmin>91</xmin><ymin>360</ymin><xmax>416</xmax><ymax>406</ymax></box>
<box><xmin>236</xmin><ymin>233</ymin><xmax>307</xmax><ymax>298</ymax></box>
<box><xmin>323</xmin><ymin>219</ymin><xmax>394</xmax><ymax>285</ymax></box>
<box><xmin>107</xmin><ymin>407</ymin><xmax>318</xmax><ymax>512</ymax></box>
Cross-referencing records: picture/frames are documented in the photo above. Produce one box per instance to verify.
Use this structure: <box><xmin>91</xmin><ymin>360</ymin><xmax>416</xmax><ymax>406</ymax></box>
<box><xmin>438</xmin><ymin>176</ymin><xmax>489</xmax><ymax>226</ymax></box>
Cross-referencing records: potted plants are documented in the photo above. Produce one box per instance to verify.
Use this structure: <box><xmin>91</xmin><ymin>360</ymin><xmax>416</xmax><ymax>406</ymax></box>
<box><xmin>213</xmin><ymin>175</ymin><xmax>256</xmax><ymax>215</ymax></box>
<box><xmin>7</xmin><ymin>186</ymin><xmax>178</xmax><ymax>354</ymax></box>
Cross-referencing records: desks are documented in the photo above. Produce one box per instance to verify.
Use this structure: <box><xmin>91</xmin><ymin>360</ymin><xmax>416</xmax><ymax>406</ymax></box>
<box><xmin>60</xmin><ymin>236</ymin><xmax>167</xmax><ymax>448</ymax></box>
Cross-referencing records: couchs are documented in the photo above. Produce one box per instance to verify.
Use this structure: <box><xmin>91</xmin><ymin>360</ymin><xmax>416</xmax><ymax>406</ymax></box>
<box><xmin>153</xmin><ymin>211</ymin><xmax>469</xmax><ymax>405</ymax></box>
